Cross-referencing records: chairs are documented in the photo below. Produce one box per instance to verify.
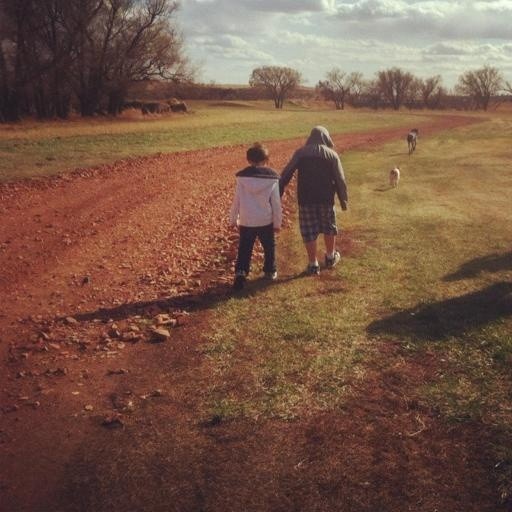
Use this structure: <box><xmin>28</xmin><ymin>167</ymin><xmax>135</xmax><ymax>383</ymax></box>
<box><xmin>235</xmin><ymin>270</ymin><xmax>245</xmax><ymax>289</ymax></box>
<box><xmin>324</xmin><ymin>251</ymin><xmax>341</xmax><ymax>269</ymax></box>
<box><xmin>263</xmin><ymin>271</ymin><xmax>278</xmax><ymax>281</ymax></box>
<box><xmin>306</xmin><ymin>263</ymin><xmax>320</xmax><ymax>275</ymax></box>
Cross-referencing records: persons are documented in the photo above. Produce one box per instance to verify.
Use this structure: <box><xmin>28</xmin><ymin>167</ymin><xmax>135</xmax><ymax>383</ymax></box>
<box><xmin>229</xmin><ymin>145</ymin><xmax>282</xmax><ymax>289</ymax></box>
<box><xmin>280</xmin><ymin>125</ymin><xmax>349</xmax><ymax>275</ymax></box>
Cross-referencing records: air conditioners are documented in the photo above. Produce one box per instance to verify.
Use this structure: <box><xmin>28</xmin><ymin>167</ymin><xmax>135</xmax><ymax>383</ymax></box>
<box><xmin>389</xmin><ymin>166</ymin><xmax>400</xmax><ymax>189</ymax></box>
<box><xmin>406</xmin><ymin>128</ymin><xmax>419</xmax><ymax>155</ymax></box>
